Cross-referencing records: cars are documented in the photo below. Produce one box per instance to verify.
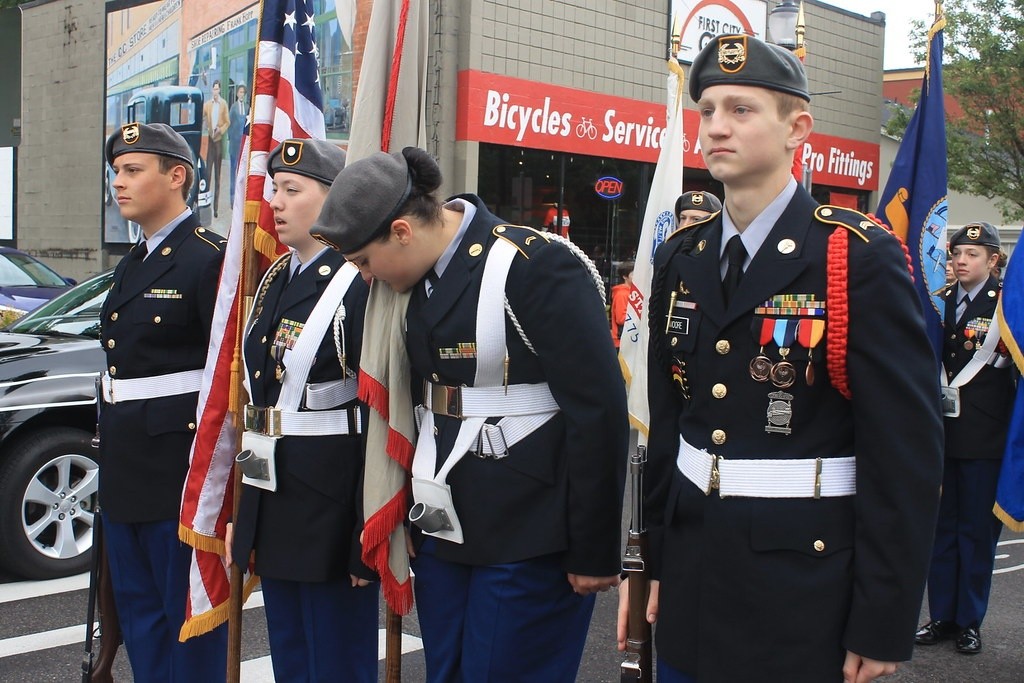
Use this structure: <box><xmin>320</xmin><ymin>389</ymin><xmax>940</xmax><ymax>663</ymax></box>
<box><xmin>0</xmin><ymin>267</ymin><xmax>124</xmax><ymax>579</ymax></box>
<box><xmin>0</xmin><ymin>246</ymin><xmax>79</xmax><ymax>319</ymax></box>
<box><xmin>105</xmin><ymin>86</ymin><xmax>212</xmax><ymax>244</ymax></box>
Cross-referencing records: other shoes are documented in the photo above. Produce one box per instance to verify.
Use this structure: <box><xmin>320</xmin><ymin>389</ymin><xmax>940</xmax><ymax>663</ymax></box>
<box><xmin>214</xmin><ymin>209</ymin><xmax>217</xmax><ymax>217</ymax></box>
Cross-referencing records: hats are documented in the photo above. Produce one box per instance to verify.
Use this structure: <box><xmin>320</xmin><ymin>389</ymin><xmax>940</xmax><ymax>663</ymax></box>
<box><xmin>946</xmin><ymin>250</ymin><xmax>952</xmax><ymax>260</ymax></box>
<box><xmin>105</xmin><ymin>123</ymin><xmax>195</xmax><ymax>170</ymax></box>
<box><xmin>237</xmin><ymin>80</ymin><xmax>249</xmax><ymax>94</ymax></box>
<box><xmin>674</xmin><ymin>190</ymin><xmax>723</xmax><ymax>224</ymax></box>
<box><xmin>267</xmin><ymin>138</ymin><xmax>347</xmax><ymax>185</ymax></box>
<box><xmin>949</xmin><ymin>223</ymin><xmax>1001</xmax><ymax>254</ymax></box>
<box><xmin>308</xmin><ymin>151</ymin><xmax>412</xmax><ymax>253</ymax></box>
<box><xmin>689</xmin><ymin>33</ymin><xmax>812</xmax><ymax>104</ymax></box>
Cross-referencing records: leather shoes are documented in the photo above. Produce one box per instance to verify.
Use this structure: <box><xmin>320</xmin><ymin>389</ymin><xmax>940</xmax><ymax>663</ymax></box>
<box><xmin>954</xmin><ymin>628</ymin><xmax>981</xmax><ymax>653</ymax></box>
<box><xmin>915</xmin><ymin>617</ymin><xmax>956</xmax><ymax>645</ymax></box>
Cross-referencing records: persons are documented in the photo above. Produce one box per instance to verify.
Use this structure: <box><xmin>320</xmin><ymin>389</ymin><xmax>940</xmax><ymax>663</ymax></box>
<box><xmin>203</xmin><ymin>80</ymin><xmax>230</xmax><ymax>217</ymax></box>
<box><xmin>227</xmin><ymin>85</ymin><xmax>250</xmax><ymax>209</ymax></box>
<box><xmin>96</xmin><ymin>33</ymin><xmax>1024</xmax><ymax>683</ymax></box>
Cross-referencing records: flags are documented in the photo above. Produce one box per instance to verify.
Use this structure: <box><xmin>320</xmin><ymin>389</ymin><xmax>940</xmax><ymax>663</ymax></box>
<box><xmin>875</xmin><ymin>14</ymin><xmax>948</xmax><ymax>377</ymax></box>
<box><xmin>992</xmin><ymin>228</ymin><xmax>1024</xmax><ymax>531</ymax></box>
<box><xmin>177</xmin><ymin>0</ymin><xmax>327</xmax><ymax>645</ymax></box>
<box><xmin>617</xmin><ymin>58</ymin><xmax>683</xmax><ymax>453</ymax></box>
<box><xmin>346</xmin><ymin>0</ymin><xmax>429</xmax><ymax>617</ymax></box>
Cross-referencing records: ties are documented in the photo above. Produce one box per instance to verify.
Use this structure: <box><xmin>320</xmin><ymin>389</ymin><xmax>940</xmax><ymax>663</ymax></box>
<box><xmin>241</xmin><ymin>100</ymin><xmax>244</xmax><ymax>115</ymax></box>
<box><xmin>721</xmin><ymin>235</ymin><xmax>748</xmax><ymax>299</ymax></box>
<box><xmin>119</xmin><ymin>241</ymin><xmax>148</xmax><ymax>298</ymax></box>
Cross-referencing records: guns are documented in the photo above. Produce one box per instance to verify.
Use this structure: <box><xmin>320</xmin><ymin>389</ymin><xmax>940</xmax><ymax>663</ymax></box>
<box><xmin>81</xmin><ymin>371</ymin><xmax>123</xmax><ymax>683</ymax></box>
<box><xmin>621</xmin><ymin>444</ymin><xmax>652</xmax><ymax>683</ymax></box>
